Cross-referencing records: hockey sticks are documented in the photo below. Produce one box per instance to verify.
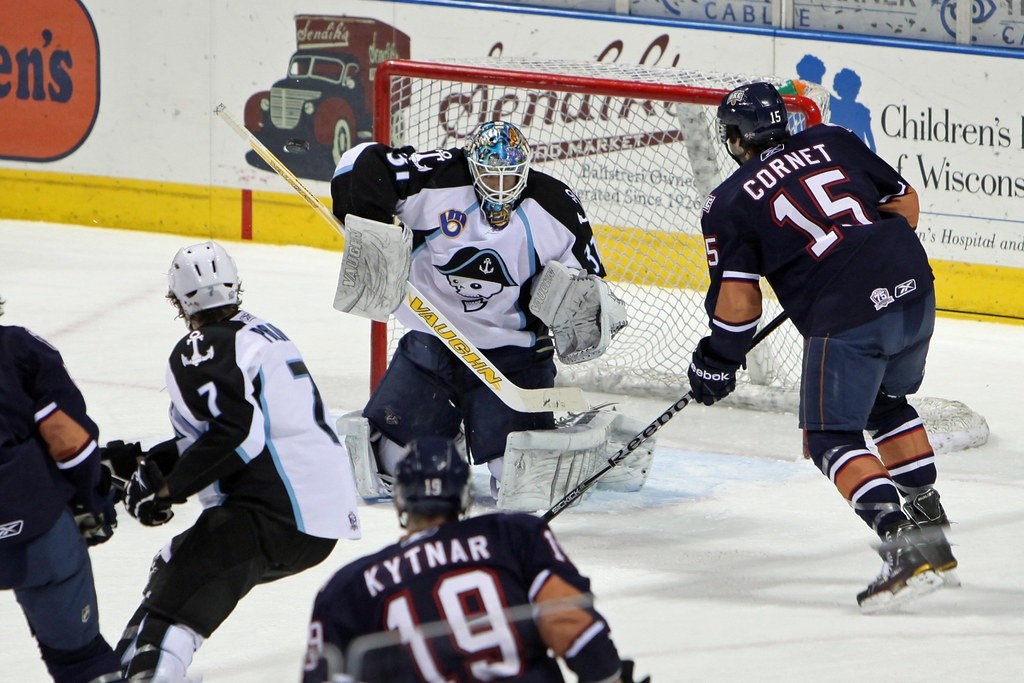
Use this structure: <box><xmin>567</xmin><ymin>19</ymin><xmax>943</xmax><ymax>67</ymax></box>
<box><xmin>79</xmin><ymin>517</ymin><xmax>96</xmax><ymax>535</ymax></box>
<box><xmin>540</xmin><ymin>309</ymin><xmax>790</xmax><ymax>527</ymax></box>
<box><xmin>111</xmin><ymin>474</ymin><xmax>172</xmax><ymax>526</ymax></box>
<box><xmin>212</xmin><ymin>101</ymin><xmax>587</xmax><ymax>416</ymax></box>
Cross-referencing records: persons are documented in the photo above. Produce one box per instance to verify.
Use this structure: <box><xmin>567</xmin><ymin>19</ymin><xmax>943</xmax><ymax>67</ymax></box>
<box><xmin>687</xmin><ymin>82</ymin><xmax>963</xmax><ymax>617</ymax></box>
<box><xmin>300</xmin><ymin>434</ymin><xmax>652</xmax><ymax>682</ymax></box>
<box><xmin>0</xmin><ymin>299</ymin><xmax>126</xmax><ymax>682</ymax></box>
<box><xmin>108</xmin><ymin>241</ymin><xmax>362</xmax><ymax>683</ymax></box>
<box><xmin>330</xmin><ymin>119</ymin><xmax>628</xmax><ymax>506</ymax></box>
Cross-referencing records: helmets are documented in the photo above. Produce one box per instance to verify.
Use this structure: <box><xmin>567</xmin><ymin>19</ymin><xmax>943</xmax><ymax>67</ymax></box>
<box><xmin>168</xmin><ymin>240</ymin><xmax>244</xmax><ymax>316</ymax></box>
<box><xmin>717</xmin><ymin>81</ymin><xmax>789</xmax><ymax>145</ymax></box>
<box><xmin>465</xmin><ymin>119</ymin><xmax>532</xmax><ymax>230</ymax></box>
<box><xmin>393</xmin><ymin>437</ymin><xmax>474</xmax><ymax>515</ymax></box>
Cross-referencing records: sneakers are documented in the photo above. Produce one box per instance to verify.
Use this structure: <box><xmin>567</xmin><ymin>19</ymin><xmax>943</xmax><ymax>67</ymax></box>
<box><xmin>854</xmin><ymin>489</ymin><xmax>962</xmax><ymax>615</ymax></box>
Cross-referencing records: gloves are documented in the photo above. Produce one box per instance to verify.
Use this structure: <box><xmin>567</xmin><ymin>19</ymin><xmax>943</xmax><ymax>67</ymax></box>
<box><xmin>101</xmin><ymin>439</ymin><xmax>174</xmax><ymax>526</ymax></box>
<box><xmin>69</xmin><ymin>494</ymin><xmax>117</xmax><ymax>548</ymax></box>
<box><xmin>688</xmin><ymin>335</ymin><xmax>748</xmax><ymax>407</ymax></box>
